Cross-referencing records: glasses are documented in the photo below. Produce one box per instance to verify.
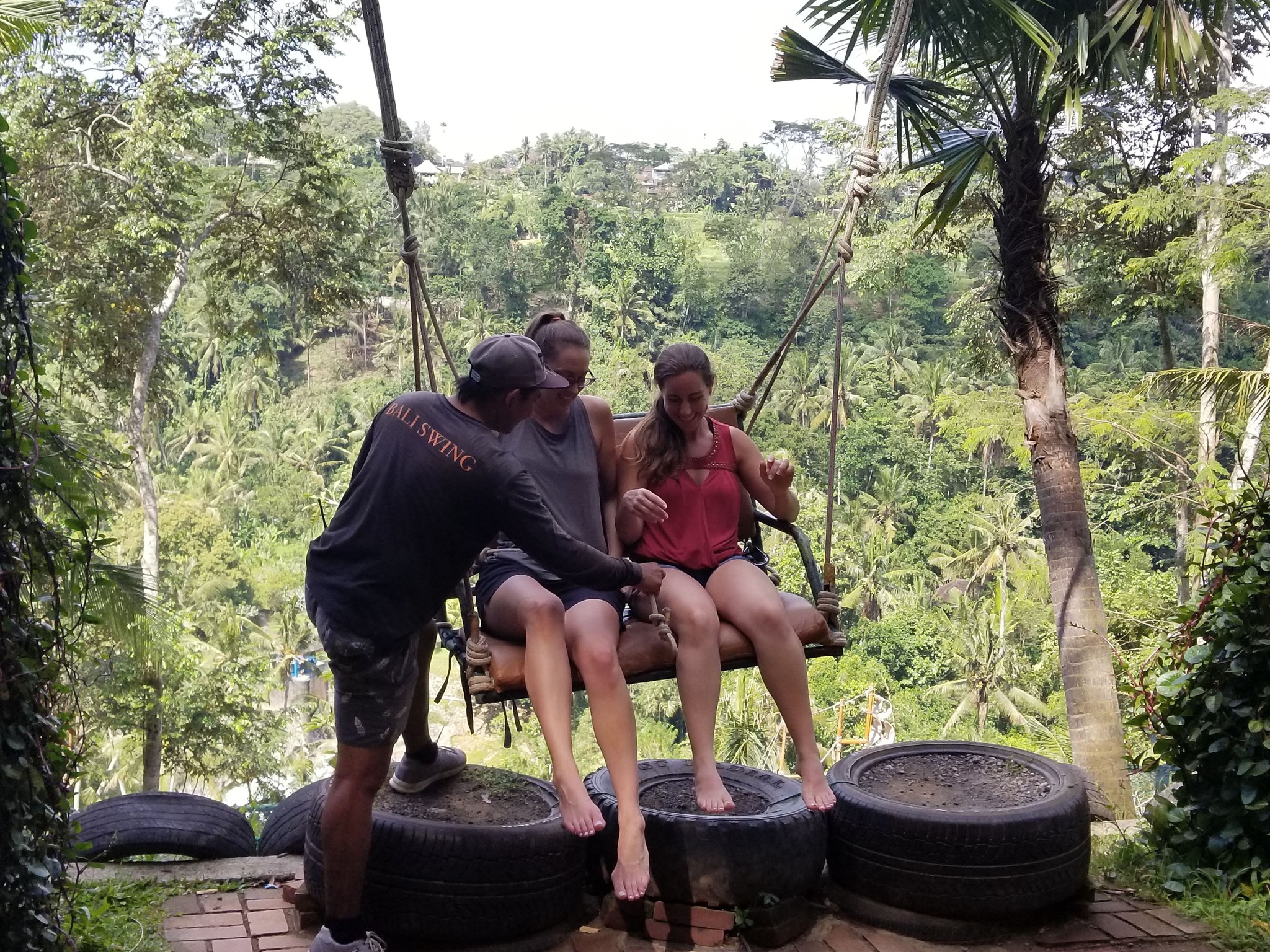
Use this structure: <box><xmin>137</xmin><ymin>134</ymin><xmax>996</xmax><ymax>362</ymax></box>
<box><xmin>564</xmin><ymin>368</ymin><xmax>596</xmax><ymax>387</ymax></box>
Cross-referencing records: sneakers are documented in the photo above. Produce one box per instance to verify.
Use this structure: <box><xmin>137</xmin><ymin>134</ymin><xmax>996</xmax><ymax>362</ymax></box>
<box><xmin>307</xmin><ymin>924</ymin><xmax>387</xmax><ymax>952</ymax></box>
<box><xmin>389</xmin><ymin>742</ymin><xmax>467</xmax><ymax>792</ymax></box>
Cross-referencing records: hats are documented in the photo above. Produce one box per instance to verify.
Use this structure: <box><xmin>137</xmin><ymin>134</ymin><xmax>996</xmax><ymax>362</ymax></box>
<box><xmin>467</xmin><ymin>332</ymin><xmax>570</xmax><ymax>389</ymax></box>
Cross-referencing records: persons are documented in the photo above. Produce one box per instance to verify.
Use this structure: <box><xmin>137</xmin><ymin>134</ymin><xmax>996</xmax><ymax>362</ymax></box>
<box><xmin>476</xmin><ymin>312</ymin><xmax>651</xmax><ymax>901</ymax></box>
<box><xmin>304</xmin><ymin>334</ymin><xmax>667</xmax><ymax>952</ymax></box>
<box><xmin>614</xmin><ymin>344</ymin><xmax>838</xmax><ymax>813</ymax></box>
